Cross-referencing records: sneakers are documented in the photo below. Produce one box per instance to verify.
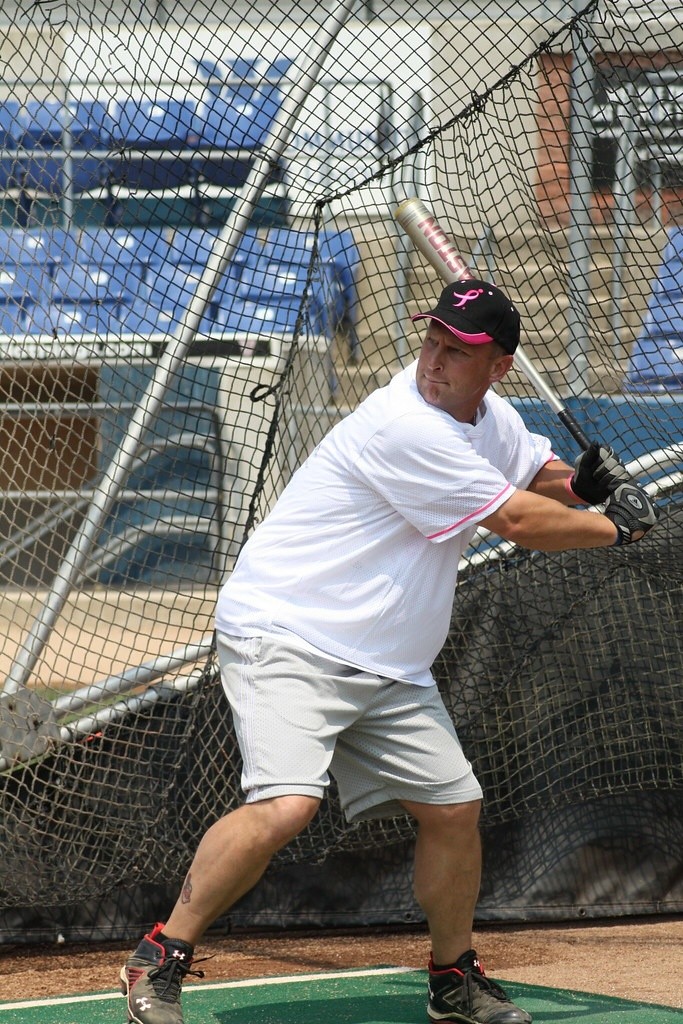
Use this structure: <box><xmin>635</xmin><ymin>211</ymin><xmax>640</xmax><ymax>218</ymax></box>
<box><xmin>425</xmin><ymin>949</ymin><xmax>533</xmax><ymax>1023</ymax></box>
<box><xmin>119</xmin><ymin>924</ymin><xmax>214</xmax><ymax>1024</ymax></box>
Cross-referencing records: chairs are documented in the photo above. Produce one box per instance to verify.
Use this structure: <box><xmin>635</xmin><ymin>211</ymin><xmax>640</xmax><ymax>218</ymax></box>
<box><xmin>0</xmin><ymin>98</ymin><xmax>365</xmax><ymax>403</ymax></box>
<box><xmin>622</xmin><ymin>228</ymin><xmax>683</xmax><ymax>394</ymax></box>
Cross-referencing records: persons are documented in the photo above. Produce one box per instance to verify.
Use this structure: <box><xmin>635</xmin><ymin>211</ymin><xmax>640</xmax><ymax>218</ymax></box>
<box><xmin>121</xmin><ymin>280</ymin><xmax>661</xmax><ymax>1024</ymax></box>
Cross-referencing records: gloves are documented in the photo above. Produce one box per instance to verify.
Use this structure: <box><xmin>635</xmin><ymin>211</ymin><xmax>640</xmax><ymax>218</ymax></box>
<box><xmin>603</xmin><ymin>477</ymin><xmax>660</xmax><ymax>547</ymax></box>
<box><xmin>565</xmin><ymin>439</ymin><xmax>631</xmax><ymax>507</ymax></box>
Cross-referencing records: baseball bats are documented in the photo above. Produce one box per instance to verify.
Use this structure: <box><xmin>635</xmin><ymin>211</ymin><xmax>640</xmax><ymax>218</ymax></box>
<box><xmin>387</xmin><ymin>191</ymin><xmax>655</xmax><ymax>530</ymax></box>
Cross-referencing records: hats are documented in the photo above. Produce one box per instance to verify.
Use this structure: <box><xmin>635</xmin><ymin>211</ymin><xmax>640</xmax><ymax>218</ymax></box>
<box><xmin>412</xmin><ymin>277</ymin><xmax>521</xmax><ymax>354</ymax></box>
